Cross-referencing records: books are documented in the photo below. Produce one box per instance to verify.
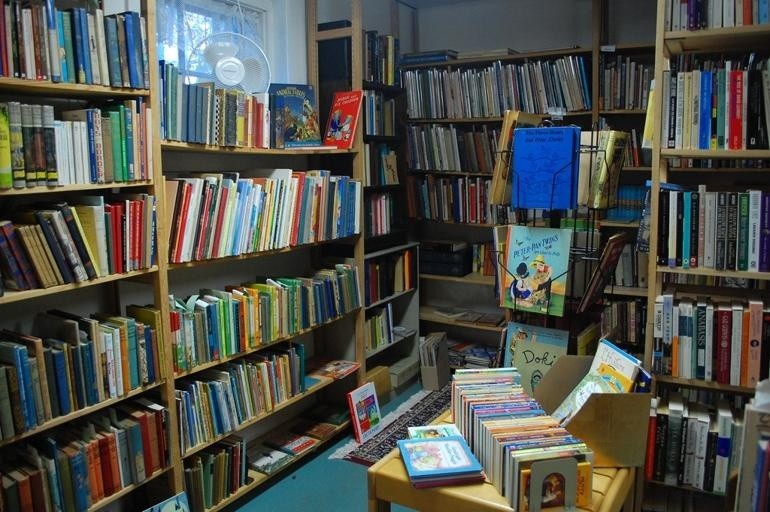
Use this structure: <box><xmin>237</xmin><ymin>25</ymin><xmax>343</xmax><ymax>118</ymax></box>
<box><xmin>656</xmin><ymin>2</ymin><xmax>768</xmax><ymax>512</ymax></box>
<box><xmin>0</xmin><ymin>0</ymin><xmax>421</xmax><ymax>511</ymax></box>
<box><xmin>402</xmin><ymin>41</ymin><xmax>653</xmax><ymax>512</ymax></box>
